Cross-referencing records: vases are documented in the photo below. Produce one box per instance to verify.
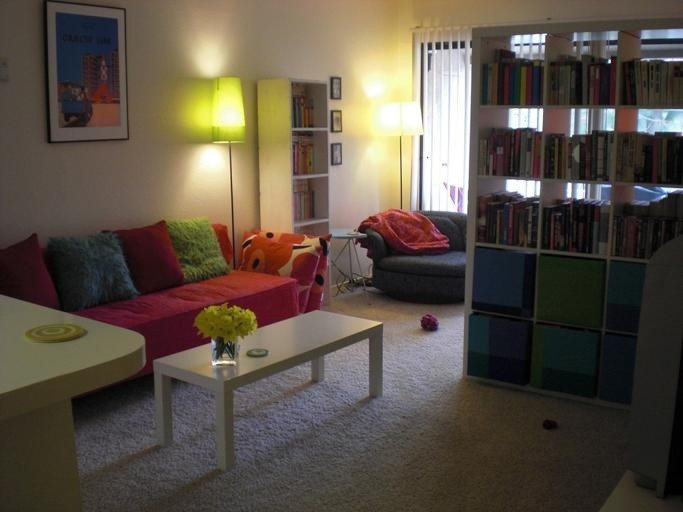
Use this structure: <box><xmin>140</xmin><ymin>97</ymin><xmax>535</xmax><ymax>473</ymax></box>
<box><xmin>210</xmin><ymin>336</ymin><xmax>240</xmax><ymax>368</ymax></box>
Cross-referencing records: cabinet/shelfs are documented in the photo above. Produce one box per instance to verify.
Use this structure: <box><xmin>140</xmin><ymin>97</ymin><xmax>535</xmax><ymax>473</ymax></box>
<box><xmin>257</xmin><ymin>77</ymin><xmax>334</xmax><ymax>235</ymax></box>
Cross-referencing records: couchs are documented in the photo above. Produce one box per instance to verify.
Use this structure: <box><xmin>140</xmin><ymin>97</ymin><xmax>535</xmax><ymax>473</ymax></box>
<box><xmin>40</xmin><ymin>224</ymin><xmax>300</xmax><ymax>382</ymax></box>
<box><xmin>365</xmin><ymin>211</ymin><xmax>467</xmax><ymax>305</ymax></box>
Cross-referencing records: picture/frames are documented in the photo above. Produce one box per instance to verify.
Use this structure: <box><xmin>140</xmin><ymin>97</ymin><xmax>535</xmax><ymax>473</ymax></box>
<box><xmin>331</xmin><ymin>110</ymin><xmax>342</xmax><ymax>132</ymax></box>
<box><xmin>43</xmin><ymin>0</ymin><xmax>129</xmax><ymax>144</ymax></box>
<box><xmin>331</xmin><ymin>143</ymin><xmax>342</xmax><ymax>165</ymax></box>
<box><xmin>330</xmin><ymin>77</ymin><xmax>341</xmax><ymax>99</ymax></box>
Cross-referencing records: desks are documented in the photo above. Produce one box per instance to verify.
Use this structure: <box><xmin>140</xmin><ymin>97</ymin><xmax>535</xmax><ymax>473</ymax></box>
<box><xmin>330</xmin><ymin>228</ymin><xmax>373</xmax><ymax>296</ymax></box>
<box><xmin>152</xmin><ymin>309</ymin><xmax>383</xmax><ymax>472</ymax></box>
<box><xmin>0</xmin><ymin>294</ymin><xmax>146</xmax><ymax>512</ymax></box>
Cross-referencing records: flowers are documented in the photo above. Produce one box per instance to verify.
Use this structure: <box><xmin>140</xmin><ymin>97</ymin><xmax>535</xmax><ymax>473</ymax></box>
<box><xmin>193</xmin><ymin>303</ymin><xmax>258</xmax><ymax>363</ymax></box>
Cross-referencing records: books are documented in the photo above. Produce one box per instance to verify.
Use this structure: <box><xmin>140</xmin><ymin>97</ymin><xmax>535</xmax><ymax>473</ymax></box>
<box><xmin>478</xmin><ymin>191</ymin><xmax>683</xmax><ymax>259</ymax></box>
<box><xmin>477</xmin><ymin>49</ymin><xmax>683</xmax><ymax>106</ymax></box>
<box><xmin>292</xmin><ymin>89</ymin><xmax>315</xmax><ymax>221</ymax></box>
<box><xmin>478</xmin><ymin>127</ymin><xmax>683</xmax><ymax>184</ymax></box>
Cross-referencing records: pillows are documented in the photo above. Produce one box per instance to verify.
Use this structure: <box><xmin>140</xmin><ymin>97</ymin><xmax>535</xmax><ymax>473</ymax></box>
<box><xmin>44</xmin><ymin>230</ymin><xmax>141</xmax><ymax>312</ymax></box>
<box><xmin>237</xmin><ymin>231</ymin><xmax>323</xmax><ymax>315</ymax></box>
<box><xmin>157</xmin><ymin>216</ymin><xmax>231</xmax><ymax>283</ymax></box>
<box><xmin>0</xmin><ymin>232</ymin><xmax>59</xmax><ymax>308</ymax></box>
<box><xmin>101</xmin><ymin>220</ymin><xmax>184</xmax><ymax>295</ymax></box>
<box><xmin>252</xmin><ymin>228</ymin><xmax>332</xmax><ymax>314</ymax></box>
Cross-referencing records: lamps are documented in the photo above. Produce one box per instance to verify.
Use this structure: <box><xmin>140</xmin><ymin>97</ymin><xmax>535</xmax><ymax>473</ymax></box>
<box><xmin>379</xmin><ymin>101</ymin><xmax>425</xmax><ymax>210</ymax></box>
<box><xmin>210</xmin><ymin>76</ymin><xmax>246</xmax><ymax>269</ymax></box>
